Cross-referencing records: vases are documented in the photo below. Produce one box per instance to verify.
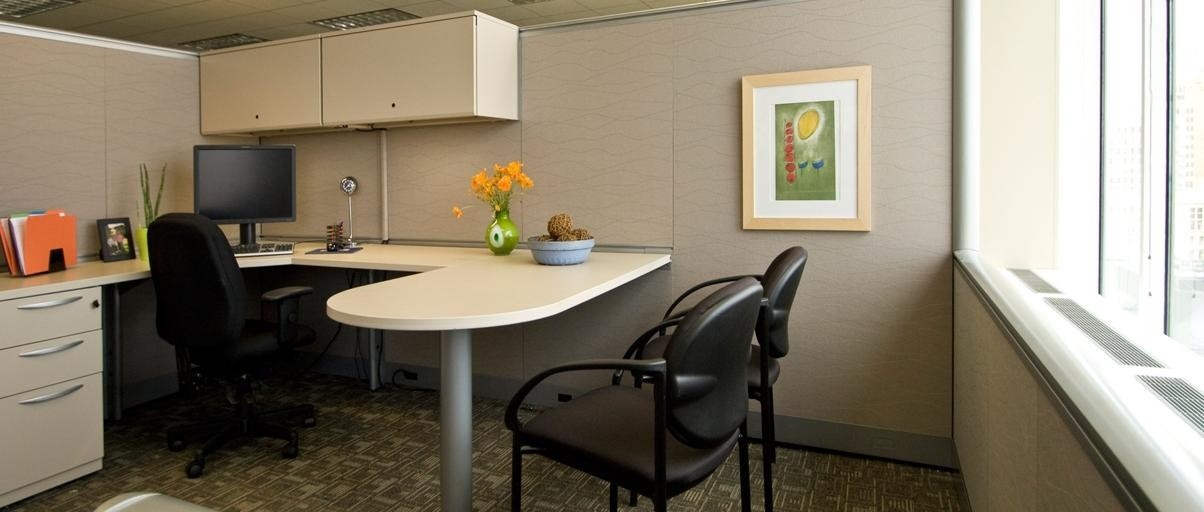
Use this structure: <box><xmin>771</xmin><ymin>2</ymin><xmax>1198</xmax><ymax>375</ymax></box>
<box><xmin>486</xmin><ymin>200</ymin><xmax>518</xmax><ymax>254</ymax></box>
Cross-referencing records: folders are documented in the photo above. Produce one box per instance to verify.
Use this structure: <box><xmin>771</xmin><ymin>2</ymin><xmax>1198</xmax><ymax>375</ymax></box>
<box><xmin>0</xmin><ymin>209</ymin><xmax>76</xmax><ymax>276</ymax></box>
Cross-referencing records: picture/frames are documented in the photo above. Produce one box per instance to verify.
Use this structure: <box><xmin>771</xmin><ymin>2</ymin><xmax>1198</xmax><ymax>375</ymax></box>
<box><xmin>94</xmin><ymin>215</ymin><xmax>137</xmax><ymax>265</ymax></box>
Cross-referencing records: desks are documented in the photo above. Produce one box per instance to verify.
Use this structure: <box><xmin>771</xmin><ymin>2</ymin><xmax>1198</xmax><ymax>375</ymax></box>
<box><xmin>0</xmin><ymin>242</ymin><xmax>672</xmax><ymax>510</ymax></box>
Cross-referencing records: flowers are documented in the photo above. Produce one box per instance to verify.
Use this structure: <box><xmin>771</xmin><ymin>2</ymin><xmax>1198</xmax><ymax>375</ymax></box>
<box><xmin>446</xmin><ymin>156</ymin><xmax>535</xmax><ymax>220</ymax></box>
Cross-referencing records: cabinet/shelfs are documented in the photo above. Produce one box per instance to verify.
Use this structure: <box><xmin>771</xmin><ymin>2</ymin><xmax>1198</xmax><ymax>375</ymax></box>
<box><xmin>1</xmin><ymin>283</ymin><xmax>107</xmax><ymax>505</ymax></box>
<box><xmin>199</xmin><ymin>32</ymin><xmax>320</xmax><ymax>136</ymax></box>
<box><xmin>323</xmin><ymin>11</ymin><xmax>517</xmax><ymax>130</ymax></box>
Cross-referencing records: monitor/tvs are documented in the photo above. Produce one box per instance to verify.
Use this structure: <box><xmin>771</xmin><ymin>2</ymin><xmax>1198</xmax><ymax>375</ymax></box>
<box><xmin>192</xmin><ymin>145</ymin><xmax>296</xmax><ymax>245</ymax></box>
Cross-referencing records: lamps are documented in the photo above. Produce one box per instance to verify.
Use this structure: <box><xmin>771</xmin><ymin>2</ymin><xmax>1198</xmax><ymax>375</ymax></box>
<box><xmin>739</xmin><ymin>63</ymin><xmax>874</xmax><ymax>237</ymax></box>
<box><xmin>338</xmin><ymin>172</ymin><xmax>364</xmax><ymax>250</ymax></box>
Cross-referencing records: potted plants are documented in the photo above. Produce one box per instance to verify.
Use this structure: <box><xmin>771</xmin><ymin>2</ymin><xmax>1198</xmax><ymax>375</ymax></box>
<box><xmin>134</xmin><ymin>161</ymin><xmax>170</xmax><ymax>264</ymax></box>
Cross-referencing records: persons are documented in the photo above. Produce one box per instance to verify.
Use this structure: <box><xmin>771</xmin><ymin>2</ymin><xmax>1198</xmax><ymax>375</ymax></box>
<box><xmin>116</xmin><ymin>228</ymin><xmax>124</xmax><ymax>250</ymax></box>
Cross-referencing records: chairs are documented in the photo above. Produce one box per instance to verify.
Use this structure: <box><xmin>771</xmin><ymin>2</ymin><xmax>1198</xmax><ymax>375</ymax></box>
<box><xmin>503</xmin><ymin>275</ymin><xmax>764</xmax><ymax>509</ymax></box>
<box><xmin>143</xmin><ymin>210</ymin><xmax>317</xmax><ymax>477</ymax></box>
<box><xmin>627</xmin><ymin>244</ymin><xmax>808</xmax><ymax>512</ymax></box>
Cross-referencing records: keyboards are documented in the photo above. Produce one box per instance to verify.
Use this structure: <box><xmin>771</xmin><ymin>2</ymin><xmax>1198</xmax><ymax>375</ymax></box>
<box><xmin>229</xmin><ymin>242</ymin><xmax>294</xmax><ymax>258</ymax></box>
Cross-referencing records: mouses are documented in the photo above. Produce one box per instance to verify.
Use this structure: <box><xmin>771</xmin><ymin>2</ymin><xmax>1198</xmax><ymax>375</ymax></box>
<box><xmin>326</xmin><ymin>242</ymin><xmax>337</xmax><ymax>252</ymax></box>
